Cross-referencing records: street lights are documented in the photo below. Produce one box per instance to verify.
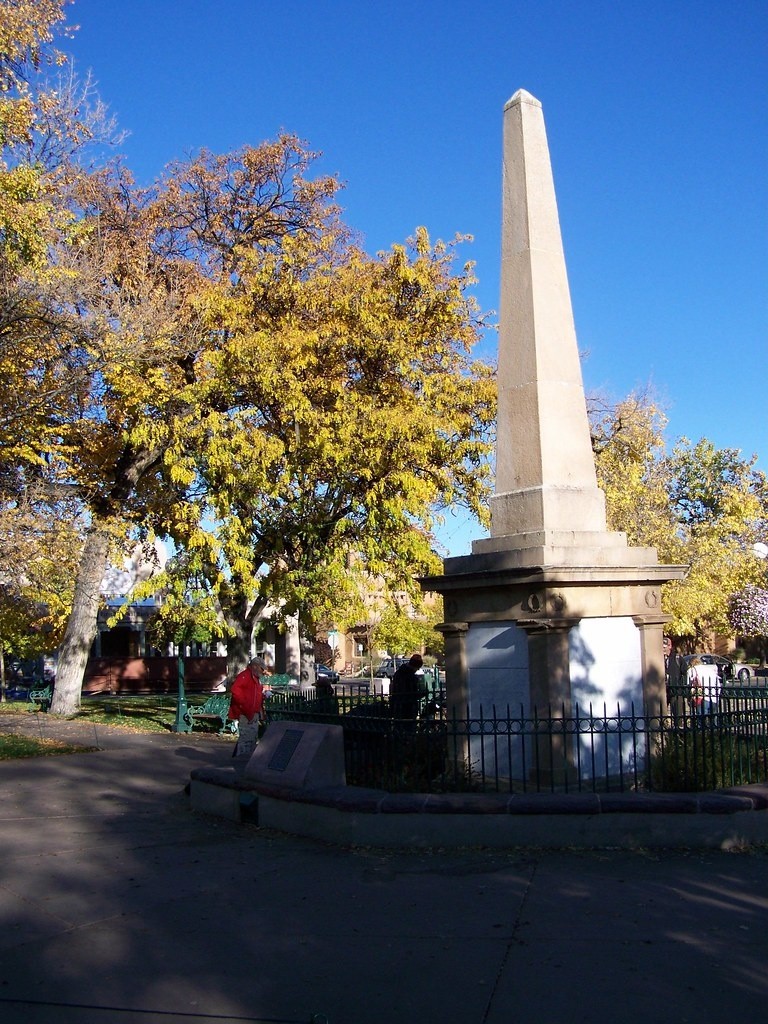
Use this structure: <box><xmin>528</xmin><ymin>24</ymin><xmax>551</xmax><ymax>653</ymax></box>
<box><xmin>165</xmin><ymin>549</ymin><xmax>195</xmax><ymax>734</ymax></box>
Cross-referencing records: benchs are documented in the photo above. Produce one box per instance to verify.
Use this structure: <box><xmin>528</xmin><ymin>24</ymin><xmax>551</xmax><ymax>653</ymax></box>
<box><xmin>184</xmin><ymin>672</ymin><xmax>291</xmax><ymax>737</ymax></box>
<box><xmin>27</xmin><ymin>683</ymin><xmax>54</xmax><ymax>706</ymax></box>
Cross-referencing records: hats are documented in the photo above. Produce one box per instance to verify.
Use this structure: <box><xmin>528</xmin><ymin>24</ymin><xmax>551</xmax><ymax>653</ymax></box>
<box><xmin>410</xmin><ymin>654</ymin><xmax>424</xmax><ymax>668</ymax></box>
<box><xmin>250</xmin><ymin>657</ymin><xmax>268</xmax><ymax>669</ymax></box>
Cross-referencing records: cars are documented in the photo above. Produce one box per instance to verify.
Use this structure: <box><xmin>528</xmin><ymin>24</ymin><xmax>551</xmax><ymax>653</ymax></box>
<box><xmin>377</xmin><ymin>658</ymin><xmax>434</xmax><ymax>679</ymax></box>
<box><xmin>678</xmin><ymin>654</ymin><xmax>755</xmax><ymax>682</ymax></box>
<box><xmin>314</xmin><ymin>663</ymin><xmax>340</xmax><ymax>685</ymax></box>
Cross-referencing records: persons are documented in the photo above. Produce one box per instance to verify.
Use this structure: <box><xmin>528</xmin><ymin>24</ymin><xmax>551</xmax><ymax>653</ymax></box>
<box><xmin>228</xmin><ymin>657</ymin><xmax>267</xmax><ymax>757</ymax></box>
<box><xmin>663</xmin><ymin>638</ymin><xmax>688</xmax><ymax>730</ymax></box>
<box><xmin>687</xmin><ymin>658</ymin><xmax>721</xmax><ymax>731</ymax></box>
<box><xmin>390</xmin><ymin>654</ymin><xmax>429</xmax><ymax>738</ymax></box>
